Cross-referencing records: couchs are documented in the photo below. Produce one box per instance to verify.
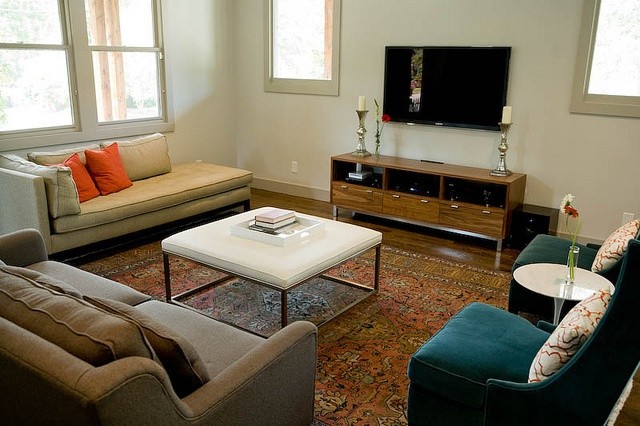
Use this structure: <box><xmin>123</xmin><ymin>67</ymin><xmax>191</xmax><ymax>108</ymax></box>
<box><xmin>509</xmin><ymin>234</ymin><xmax>639</xmax><ymax>319</ymax></box>
<box><xmin>1</xmin><ymin>229</ymin><xmax>317</xmax><ymax>426</ymax></box>
<box><xmin>407</xmin><ymin>242</ymin><xmax>639</xmax><ymax>426</ymax></box>
<box><xmin>1</xmin><ymin>134</ymin><xmax>253</xmax><ymax>256</ymax></box>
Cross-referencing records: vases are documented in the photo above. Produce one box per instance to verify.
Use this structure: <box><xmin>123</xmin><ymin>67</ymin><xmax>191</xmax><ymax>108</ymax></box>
<box><xmin>374</xmin><ymin>145</ymin><xmax>380</xmax><ymax>161</ymax></box>
<box><xmin>564</xmin><ymin>245</ymin><xmax>580</xmax><ymax>284</ymax></box>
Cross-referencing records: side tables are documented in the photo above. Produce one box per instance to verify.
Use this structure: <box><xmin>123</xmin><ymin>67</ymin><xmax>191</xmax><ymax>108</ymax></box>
<box><xmin>513</xmin><ymin>263</ymin><xmax>616</xmax><ymax>326</ymax></box>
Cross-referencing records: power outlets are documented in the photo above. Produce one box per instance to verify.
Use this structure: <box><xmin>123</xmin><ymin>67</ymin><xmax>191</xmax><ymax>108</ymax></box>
<box><xmin>290</xmin><ymin>161</ymin><xmax>298</xmax><ymax>174</ymax></box>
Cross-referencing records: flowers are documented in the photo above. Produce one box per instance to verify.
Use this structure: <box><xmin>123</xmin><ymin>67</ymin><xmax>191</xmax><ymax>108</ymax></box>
<box><xmin>373</xmin><ymin>98</ymin><xmax>391</xmax><ymax>145</ymax></box>
<box><xmin>560</xmin><ymin>193</ymin><xmax>581</xmax><ymax>248</ymax></box>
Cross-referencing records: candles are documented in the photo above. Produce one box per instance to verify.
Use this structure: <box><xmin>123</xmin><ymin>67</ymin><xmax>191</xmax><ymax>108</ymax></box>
<box><xmin>358</xmin><ymin>95</ymin><xmax>367</xmax><ymax>111</ymax></box>
<box><xmin>501</xmin><ymin>105</ymin><xmax>513</xmax><ymax>124</ymax></box>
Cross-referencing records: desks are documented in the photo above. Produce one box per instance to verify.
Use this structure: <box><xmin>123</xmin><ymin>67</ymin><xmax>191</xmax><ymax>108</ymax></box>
<box><xmin>161</xmin><ymin>207</ymin><xmax>382</xmax><ymax>339</ymax></box>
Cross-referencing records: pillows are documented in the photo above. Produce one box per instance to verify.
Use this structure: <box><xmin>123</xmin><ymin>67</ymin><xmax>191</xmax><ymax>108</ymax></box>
<box><xmin>64</xmin><ymin>154</ymin><xmax>101</xmax><ymax>203</ymax></box>
<box><xmin>528</xmin><ymin>286</ymin><xmax>611</xmax><ymax>387</ymax></box>
<box><xmin>1</xmin><ymin>259</ymin><xmax>82</xmax><ymax>299</ymax></box>
<box><xmin>85</xmin><ymin>142</ymin><xmax>133</xmax><ymax>196</ymax></box>
<box><xmin>591</xmin><ymin>220</ymin><xmax>639</xmax><ymax>272</ymax></box>
<box><xmin>83</xmin><ymin>294</ymin><xmax>210</xmax><ymax>385</ymax></box>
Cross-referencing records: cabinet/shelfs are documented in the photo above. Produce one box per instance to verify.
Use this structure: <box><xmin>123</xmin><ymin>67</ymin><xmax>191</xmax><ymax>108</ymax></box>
<box><xmin>329</xmin><ymin>149</ymin><xmax>527</xmax><ymax>252</ymax></box>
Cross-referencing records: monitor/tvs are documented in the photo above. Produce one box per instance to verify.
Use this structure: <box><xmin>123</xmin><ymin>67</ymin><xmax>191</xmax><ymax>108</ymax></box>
<box><xmin>383</xmin><ymin>45</ymin><xmax>511</xmax><ymax>131</ymax></box>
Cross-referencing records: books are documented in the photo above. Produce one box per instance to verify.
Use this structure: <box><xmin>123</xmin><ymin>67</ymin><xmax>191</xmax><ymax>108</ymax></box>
<box><xmin>255</xmin><ymin>209</ymin><xmax>296</xmax><ymax>234</ymax></box>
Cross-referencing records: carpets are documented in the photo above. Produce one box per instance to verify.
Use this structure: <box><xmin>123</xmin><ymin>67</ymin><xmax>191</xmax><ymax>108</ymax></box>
<box><xmin>63</xmin><ymin>210</ymin><xmax>513</xmax><ymax>426</ymax></box>
